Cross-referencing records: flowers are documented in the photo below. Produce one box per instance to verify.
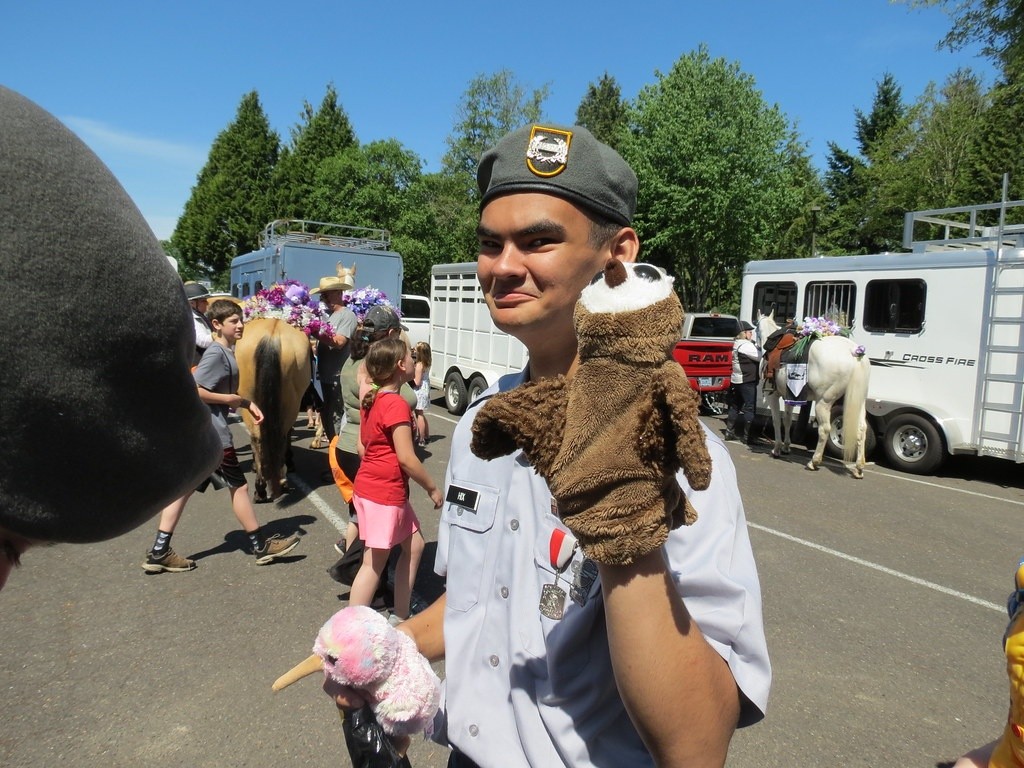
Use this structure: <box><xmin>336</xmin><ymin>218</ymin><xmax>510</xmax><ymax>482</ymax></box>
<box><xmin>237</xmin><ymin>278</ymin><xmax>400</xmax><ymax>341</ymax></box>
<box><xmin>787</xmin><ymin>316</ymin><xmax>866</xmax><ymax>360</ymax></box>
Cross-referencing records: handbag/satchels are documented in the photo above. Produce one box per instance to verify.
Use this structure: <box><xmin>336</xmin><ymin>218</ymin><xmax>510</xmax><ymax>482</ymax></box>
<box><xmin>331</xmin><ymin>534</ymin><xmax>388</xmax><ymax>597</ymax></box>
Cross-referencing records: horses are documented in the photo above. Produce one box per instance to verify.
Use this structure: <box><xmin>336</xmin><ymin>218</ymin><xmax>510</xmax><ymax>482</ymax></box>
<box><xmin>206</xmin><ymin>296</ymin><xmax>311</xmax><ymax>505</ymax></box>
<box><xmin>755</xmin><ymin>308</ymin><xmax>873</xmax><ymax>479</ymax></box>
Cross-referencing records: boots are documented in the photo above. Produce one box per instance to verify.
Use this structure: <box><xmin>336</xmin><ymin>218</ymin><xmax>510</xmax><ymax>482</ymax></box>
<box><xmin>743</xmin><ymin>421</ymin><xmax>755</xmax><ymax>445</ymax></box>
<box><xmin>725</xmin><ymin>419</ymin><xmax>741</xmax><ymax>440</ymax></box>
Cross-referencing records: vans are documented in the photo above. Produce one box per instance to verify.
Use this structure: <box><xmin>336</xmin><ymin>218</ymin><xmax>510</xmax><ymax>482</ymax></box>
<box><xmin>681</xmin><ymin>313</ymin><xmax>741</xmax><ymax>342</ymax></box>
<box><xmin>400</xmin><ymin>294</ymin><xmax>430</xmax><ymax>349</ymax></box>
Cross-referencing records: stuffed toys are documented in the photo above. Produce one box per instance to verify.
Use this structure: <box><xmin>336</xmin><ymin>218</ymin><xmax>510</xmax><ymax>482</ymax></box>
<box><xmin>473</xmin><ymin>255</ymin><xmax>711</xmax><ymax>569</ymax></box>
<box><xmin>271</xmin><ymin>607</ymin><xmax>444</xmax><ymax>742</ymax></box>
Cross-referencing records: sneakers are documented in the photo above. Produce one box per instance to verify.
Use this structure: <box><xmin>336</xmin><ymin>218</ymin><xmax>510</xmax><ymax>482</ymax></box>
<box><xmin>141</xmin><ymin>547</ymin><xmax>196</xmax><ymax>573</ymax></box>
<box><xmin>253</xmin><ymin>532</ymin><xmax>301</xmax><ymax>565</ymax></box>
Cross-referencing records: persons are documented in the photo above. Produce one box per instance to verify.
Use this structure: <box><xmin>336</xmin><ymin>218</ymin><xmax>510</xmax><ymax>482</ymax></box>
<box><xmin>300</xmin><ymin>273</ymin><xmax>361</xmax><ymax>438</ymax></box>
<box><xmin>410</xmin><ymin>341</ymin><xmax>432</xmax><ymax>448</ymax></box>
<box><xmin>349</xmin><ymin>337</ymin><xmax>445</xmax><ymax>628</ymax></box>
<box><xmin>725</xmin><ymin>320</ymin><xmax>762</xmax><ymax>445</ymax></box>
<box><xmin>333</xmin><ymin>304</ymin><xmax>418</xmax><ymax>569</ymax></box>
<box><xmin>141</xmin><ymin>298</ymin><xmax>301</xmax><ymax>573</ymax></box>
<box><xmin>389</xmin><ymin>121</ymin><xmax>776</xmax><ymax>768</ymax></box>
<box><xmin>176</xmin><ymin>281</ymin><xmax>215</xmax><ymax>375</ymax></box>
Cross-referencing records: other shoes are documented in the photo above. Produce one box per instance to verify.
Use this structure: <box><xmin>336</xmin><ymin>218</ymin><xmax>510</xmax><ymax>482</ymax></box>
<box><xmin>388</xmin><ymin>613</ymin><xmax>413</xmax><ymax>628</ymax></box>
<box><xmin>334</xmin><ymin>538</ymin><xmax>347</xmax><ymax>555</ymax></box>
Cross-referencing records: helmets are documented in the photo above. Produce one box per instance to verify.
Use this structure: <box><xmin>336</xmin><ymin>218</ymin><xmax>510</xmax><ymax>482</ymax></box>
<box><xmin>183</xmin><ymin>282</ymin><xmax>210</xmax><ymax>300</ymax></box>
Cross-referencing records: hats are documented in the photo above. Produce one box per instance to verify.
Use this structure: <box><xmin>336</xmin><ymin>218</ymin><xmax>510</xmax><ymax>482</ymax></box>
<box><xmin>475</xmin><ymin>122</ymin><xmax>638</xmax><ymax>223</ymax></box>
<box><xmin>309</xmin><ymin>276</ymin><xmax>353</xmax><ymax>296</ymax></box>
<box><xmin>735</xmin><ymin>321</ymin><xmax>756</xmax><ymax>337</ymax></box>
<box><xmin>356</xmin><ymin>305</ymin><xmax>409</xmax><ymax>333</ymax></box>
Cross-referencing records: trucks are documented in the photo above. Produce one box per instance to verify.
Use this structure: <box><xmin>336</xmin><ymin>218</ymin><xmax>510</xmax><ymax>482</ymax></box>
<box><xmin>672</xmin><ymin>342</ymin><xmax>734</xmax><ymax>395</ymax></box>
<box><xmin>229</xmin><ymin>219</ymin><xmax>402</xmax><ymax>317</ymax></box>
<box><xmin>739</xmin><ymin>172</ymin><xmax>1024</xmax><ymax>474</ymax></box>
<box><xmin>429</xmin><ymin>260</ymin><xmax>529</xmax><ymax>418</ymax></box>
